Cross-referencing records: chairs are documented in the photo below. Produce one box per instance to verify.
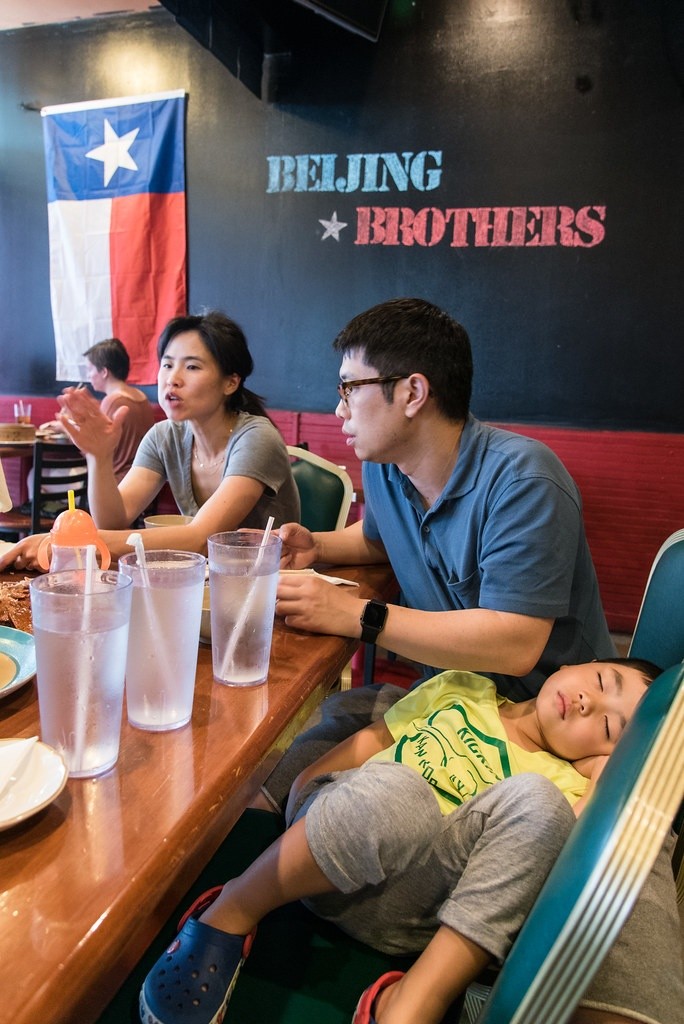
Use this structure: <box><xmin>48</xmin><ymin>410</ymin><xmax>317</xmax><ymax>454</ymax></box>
<box><xmin>225</xmin><ymin>444</ymin><xmax>684</xmax><ymax>1022</ymax></box>
<box><xmin>0</xmin><ymin>443</ymin><xmax>87</xmax><ymax>543</ymax></box>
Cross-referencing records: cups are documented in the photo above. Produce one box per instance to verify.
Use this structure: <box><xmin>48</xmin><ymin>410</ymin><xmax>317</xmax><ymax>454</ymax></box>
<box><xmin>118</xmin><ymin>550</ymin><xmax>206</xmax><ymax>732</ymax></box>
<box><xmin>27</xmin><ymin>567</ymin><xmax>134</xmax><ymax>781</ymax></box>
<box><xmin>14</xmin><ymin>404</ymin><xmax>32</xmax><ymax>424</ymax></box>
<box><xmin>207</xmin><ymin>531</ymin><xmax>283</xmax><ymax>689</ymax></box>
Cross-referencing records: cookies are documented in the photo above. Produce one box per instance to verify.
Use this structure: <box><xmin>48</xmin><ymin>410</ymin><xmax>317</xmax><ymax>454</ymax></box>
<box><xmin>0</xmin><ymin>574</ymin><xmax>41</xmax><ymax>634</ymax></box>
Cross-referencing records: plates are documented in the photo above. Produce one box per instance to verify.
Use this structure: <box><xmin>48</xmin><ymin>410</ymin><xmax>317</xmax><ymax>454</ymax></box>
<box><xmin>0</xmin><ymin>429</ymin><xmax>68</xmax><ymax>444</ymax></box>
<box><xmin>0</xmin><ymin>624</ymin><xmax>36</xmax><ymax>699</ymax></box>
<box><xmin>0</xmin><ymin>738</ymin><xmax>69</xmax><ymax>832</ymax></box>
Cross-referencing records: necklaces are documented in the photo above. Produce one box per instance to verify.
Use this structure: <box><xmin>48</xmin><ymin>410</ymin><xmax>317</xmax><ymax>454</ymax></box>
<box><xmin>193</xmin><ymin>414</ymin><xmax>233</xmax><ymax>476</ymax></box>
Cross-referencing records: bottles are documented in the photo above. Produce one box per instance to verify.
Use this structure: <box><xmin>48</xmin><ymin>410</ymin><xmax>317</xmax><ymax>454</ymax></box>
<box><xmin>37</xmin><ymin>507</ymin><xmax>112</xmax><ymax>573</ymax></box>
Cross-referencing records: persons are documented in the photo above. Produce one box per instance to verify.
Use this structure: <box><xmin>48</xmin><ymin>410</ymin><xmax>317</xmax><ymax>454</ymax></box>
<box><xmin>0</xmin><ymin>313</ymin><xmax>301</xmax><ymax>573</ymax></box>
<box><xmin>139</xmin><ymin>657</ymin><xmax>667</xmax><ymax>1024</ymax></box>
<box><xmin>236</xmin><ymin>300</ymin><xmax>684</xmax><ymax>1024</ymax></box>
<box><xmin>40</xmin><ymin>338</ymin><xmax>156</xmax><ymax>530</ymax></box>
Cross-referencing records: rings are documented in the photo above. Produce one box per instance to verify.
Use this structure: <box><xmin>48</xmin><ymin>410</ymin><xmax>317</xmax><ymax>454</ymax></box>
<box><xmin>26</xmin><ymin>566</ymin><xmax>33</xmax><ymax>570</ymax></box>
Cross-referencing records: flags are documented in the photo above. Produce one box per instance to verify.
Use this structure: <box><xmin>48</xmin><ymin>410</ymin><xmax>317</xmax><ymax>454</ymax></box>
<box><xmin>41</xmin><ymin>89</ymin><xmax>187</xmax><ymax>387</ymax></box>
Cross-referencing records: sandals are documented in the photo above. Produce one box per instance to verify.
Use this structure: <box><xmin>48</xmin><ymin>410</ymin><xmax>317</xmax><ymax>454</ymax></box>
<box><xmin>352</xmin><ymin>970</ymin><xmax>406</xmax><ymax>1024</ymax></box>
<box><xmin>140</xmin><ymin>885</ymin><xmax>257</xmax><ymax>1024</ymax></box>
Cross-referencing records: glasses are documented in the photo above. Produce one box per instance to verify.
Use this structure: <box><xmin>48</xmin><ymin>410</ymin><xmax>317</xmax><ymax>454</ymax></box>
<box><xmin>338</xmin><ymin>373</ymin><xmax>434</xmax><ymax>405</ymax></box>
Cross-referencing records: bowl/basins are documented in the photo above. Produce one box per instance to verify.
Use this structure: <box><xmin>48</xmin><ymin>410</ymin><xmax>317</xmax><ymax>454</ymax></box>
<box><xmin>144</xmin><ymin>514</ymin><xmax>195</xmax><ymax>528</ymax></box>
<box><xmin>200</xmin><ymin>585</ymin><xmax>211</xmax><ymax>639</ymax></box>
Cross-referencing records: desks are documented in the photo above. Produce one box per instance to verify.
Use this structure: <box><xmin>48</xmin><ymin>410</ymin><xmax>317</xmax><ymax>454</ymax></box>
<box><xmin>0</xmin><ymin>558</ymin><xmax>395</xmax><ymax>1024</ymax></box>
<box><xmin>0</xmin><ymin>443</ymin><xmax>56</xmax><ymax>459</ymax></box>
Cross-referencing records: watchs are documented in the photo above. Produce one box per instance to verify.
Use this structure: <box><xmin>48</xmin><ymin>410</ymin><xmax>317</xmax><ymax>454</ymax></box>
<box><xmin>360</xmin><ymin>598</ymin><xmax>389</xmax><ymax>644</ymax></box>
